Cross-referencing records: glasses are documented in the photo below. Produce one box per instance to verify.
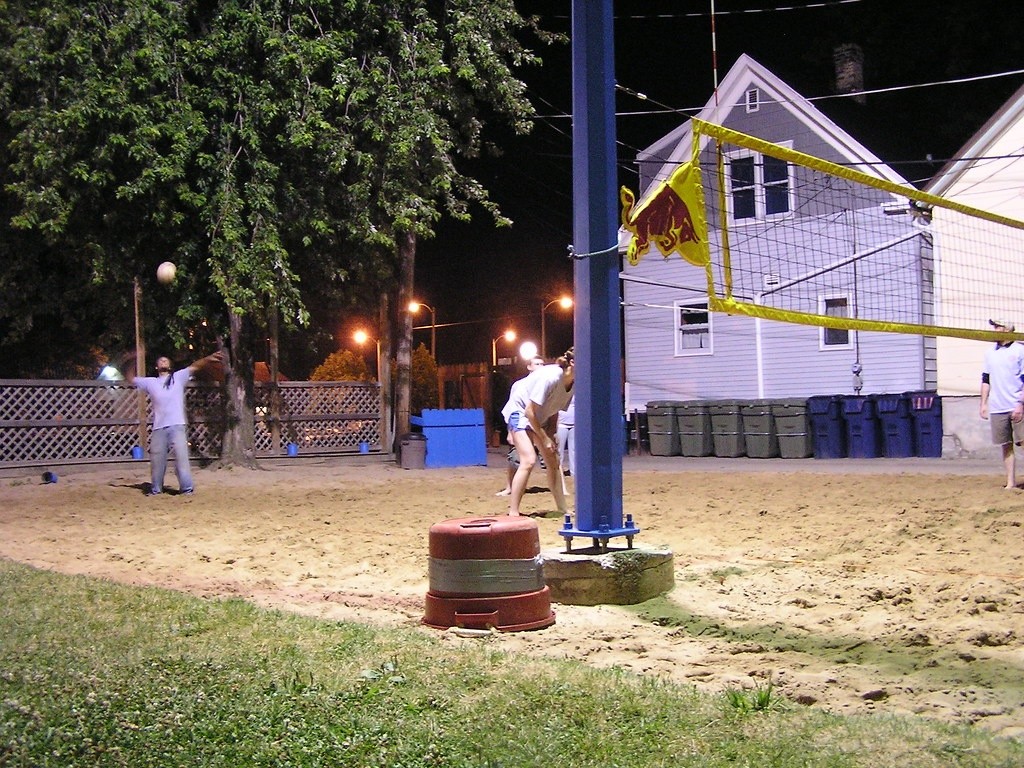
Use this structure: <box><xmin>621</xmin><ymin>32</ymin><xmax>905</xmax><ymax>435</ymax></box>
<box><xmin>994</xmin><ymin>325</ymin><xmax>1001</xmax><ymax>329</ymax></box>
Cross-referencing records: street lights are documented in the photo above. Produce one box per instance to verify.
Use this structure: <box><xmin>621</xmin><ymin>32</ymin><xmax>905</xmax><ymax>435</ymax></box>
<box><xmin>492</xmin><ymin>330</ymin><xmax>518</xmax><ymax>368</ymax></box>
<box><xmin>540</xmin><ymin>296</ymin><xmax>573</xmax><ymax>360</ymax></box>
<box><xmin>410</xmin><ymin>303</ymin><xmax>437</xmax><ymax>367</ymax></box>
<box><xmin>133</xmin><ymin>260</ymin><xmax>177</xmax><ymax>457</ymax></box>
<box><xmin>354</xmin><ymin>331</ymin><xmax>390</xmax><ymax>455</ymax></box>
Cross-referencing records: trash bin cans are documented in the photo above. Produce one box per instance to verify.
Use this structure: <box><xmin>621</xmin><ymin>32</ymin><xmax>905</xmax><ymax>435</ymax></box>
<box><xmin>646</xmin><ymin>397</ymin><xmax>812</xmax><ymax>458</ymax></box>
<box><xmin>400</xmin><ymin>432</ymin><xmax>426</xmax><ymax>470</ymax></box>
<box><xmin>806</xmin><ymin>390</ymin><xmax>942</xmax><ymax>459</ymax></box>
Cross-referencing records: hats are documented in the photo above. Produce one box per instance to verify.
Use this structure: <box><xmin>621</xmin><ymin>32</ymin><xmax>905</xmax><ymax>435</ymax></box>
<box><xmin>989</xmin><ymin>317</ymin><xmax>1015</xmax><ymax>332</ymax></box>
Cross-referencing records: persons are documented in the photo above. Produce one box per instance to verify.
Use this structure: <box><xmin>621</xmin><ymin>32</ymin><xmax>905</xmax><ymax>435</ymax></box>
<box><xmin>126</xmin><ymin>350</ymin><xmax>224</xmax><ymax>496</ymax></box>
<box><xmin>979</xmin><ymin>319</ymin><xmax>1024</xmax><ymax>490</ymax></box>
<box><xmin>496</xmin><ymin>348</ymin><xmax>576</xmax><ymax>518</ymax></box>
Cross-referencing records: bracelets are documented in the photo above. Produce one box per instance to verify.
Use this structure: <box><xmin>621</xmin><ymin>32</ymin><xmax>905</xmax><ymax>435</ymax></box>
<box><xmin>1018</xmin><ymin>400</ymin><xmax>1024</xmax><ymax>406</ymax></box>
